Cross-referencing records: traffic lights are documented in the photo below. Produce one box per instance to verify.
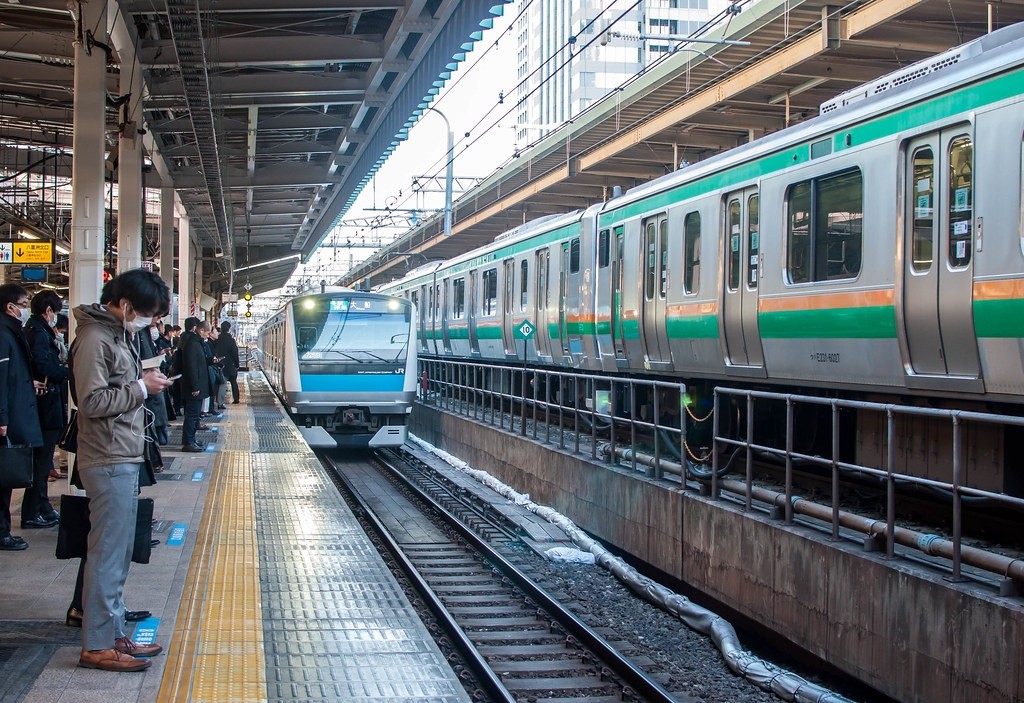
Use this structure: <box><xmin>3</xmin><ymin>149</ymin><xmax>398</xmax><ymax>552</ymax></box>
<box><xmin>244</xmin><ymin>293</ymin><xmax>251</xmax><ymax>318</ymax></box>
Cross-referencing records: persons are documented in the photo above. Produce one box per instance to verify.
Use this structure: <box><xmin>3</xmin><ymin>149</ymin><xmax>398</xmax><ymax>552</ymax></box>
<box><xmin>0</xmin><ymin>283</ymin><xmax>44</xmax><ymax>550</ymax></box>
<box><xmin>134</xmin><ymin>317</ymin><xmax>241</xmax><ymax>545</ymax></box>
<box><xmin>65</xmin><ymin>340</ymin><xmax>153</xmax><ymax>627</ymax></box>
<box><xmin>21</xmin><ymin>291</ymin><xmax>69</xmax><ymax>529</ymax></box>
<box><xmin>72</xmin><ymin>267</ymin><xmax>173</xmax><ymax>672</ymax></box>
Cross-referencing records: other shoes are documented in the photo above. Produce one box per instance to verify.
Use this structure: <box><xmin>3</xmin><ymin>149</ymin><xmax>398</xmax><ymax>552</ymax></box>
<box><xmin>196</xmin><ymin>424</ymin><xmax>207</xmax><ymax>430</ymax></box>
<box><xmin>200</xmin><ymin>404</ymin><xmax>226</xmax><ymax>419</ymax></box>
<box><xmin>182</xmin><ymin>440</ymin><xmax>204</xmax><ymax>452</ymax></box>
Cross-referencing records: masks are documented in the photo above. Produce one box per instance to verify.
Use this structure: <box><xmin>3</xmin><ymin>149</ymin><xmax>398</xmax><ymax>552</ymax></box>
<box><xmin>56</xmin><ymin>333</ymin><xmax>64</xmax><ymax>339</ymax></box>
<box><xmin>150</xmin><ymin>327</ymin><xmax>160</xmax><ymax>340</ymax></box>
<box><xmin>12</xmin><ymin>306</ymin><xmax>30</xmax><ymax>326</ymax></box>
<box><xmin>123</xmin><ymin>304</ymin><xmax>152</xmax><ymax>334</ymax></box>
<box><xmin>48</xmin><ymin>311</ymin><xmax>57</xmax><ymax>328</ymax></box>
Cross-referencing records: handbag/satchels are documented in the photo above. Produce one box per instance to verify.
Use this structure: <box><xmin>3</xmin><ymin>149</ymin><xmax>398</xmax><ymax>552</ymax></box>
<box><xmin>58</xmin><ymin>409</ymin><xmax>79</xmax><ymax>453</ymax></box>
<box><xmin>55</xmin><ymin>494</ymin><xmax>154</xmax><ymax>564</ymax></box>
<box><xmin>215</xmin><ymin>367</ymin><xmax>226</xmax><ymax>385</ymax></box>
<box><xmin>0</xmin><ymin>435</ymin><xmax>34</xmax><ymax>488</ymax></box>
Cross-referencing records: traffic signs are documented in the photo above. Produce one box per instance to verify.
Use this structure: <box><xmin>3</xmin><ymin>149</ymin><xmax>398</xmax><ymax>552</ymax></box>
<box><xmin>0</xmin><ymin>239</ymin><xmax>55</xmax><ymax>265</ymax></box>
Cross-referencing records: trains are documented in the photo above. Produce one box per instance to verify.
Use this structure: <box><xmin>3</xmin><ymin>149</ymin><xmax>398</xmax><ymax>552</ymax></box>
<box><xmin>258</xmin><ymin>278</ymin><xmax>417</xmax><ymax>449</ymax></box>
<box><xmin>370</xmin><ymin>21</ymin><xmax>1024</xmax><ymax>513</ymax></box>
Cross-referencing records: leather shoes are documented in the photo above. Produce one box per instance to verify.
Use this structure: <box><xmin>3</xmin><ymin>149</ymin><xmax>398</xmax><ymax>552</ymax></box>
<box><xmin>79</xmin><ymin>647</ymin><xmax>152</xmax><ymax>671</ymax></box>
<box><xmin>125</xmin><ymin>608</ymin><xmax>152</xmax><ymax>620</ymax></box>
<box><xmin>0</xmin><ymin>535</ymin><xmax>28</xmax><ymax>549</ymax></box>
<box><xmin>67</xmin><ymin>606</ymin><xmax>83</xmax><ymax>627</ymax></box>
<box><xmin>152</xmin><ymin>518</ymin><xmax>157</xmax><ymax>523</ymax></box>
<box><xmin>21</xmin><ymin>516</ymin><xmax>58</xmax><ymax>529</ymax></box>
<box><xmin>150</xmin><ymin>540</ymin><xmax>159</xmax><ymax>545</ymax></box>
<box><xmin>44</xmin><ymin>509</ymin><xmax>60</xmax><ymax>524</ymax></box>
<box><xmin>115</xmin><ymin>636</ymin><xmax>162</xmax><ymax>656</ymax></box>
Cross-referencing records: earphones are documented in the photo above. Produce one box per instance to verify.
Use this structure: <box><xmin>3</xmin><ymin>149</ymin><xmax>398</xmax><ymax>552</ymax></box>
<box><xmin>123</xmin><ymin>303</ymin><xmax>127</xmax><ymax>313</ymax></box>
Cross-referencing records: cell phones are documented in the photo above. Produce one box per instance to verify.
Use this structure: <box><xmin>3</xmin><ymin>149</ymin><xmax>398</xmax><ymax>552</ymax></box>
<box><xmin>218</xmin><ymin>356</ymin><xmax>225</xmax><ymax>360</ymax></box>
<box><xmin>167</xmin><ymin>374</ymin><xmax>182</xmax><ymax>381</ymax></box>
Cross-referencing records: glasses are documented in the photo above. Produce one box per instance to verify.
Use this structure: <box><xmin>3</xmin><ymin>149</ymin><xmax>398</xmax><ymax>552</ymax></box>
<box><xmin>13</xmin><ymin>301</ymin><xmax>29</xmax><ymax>308</ymax></box>
